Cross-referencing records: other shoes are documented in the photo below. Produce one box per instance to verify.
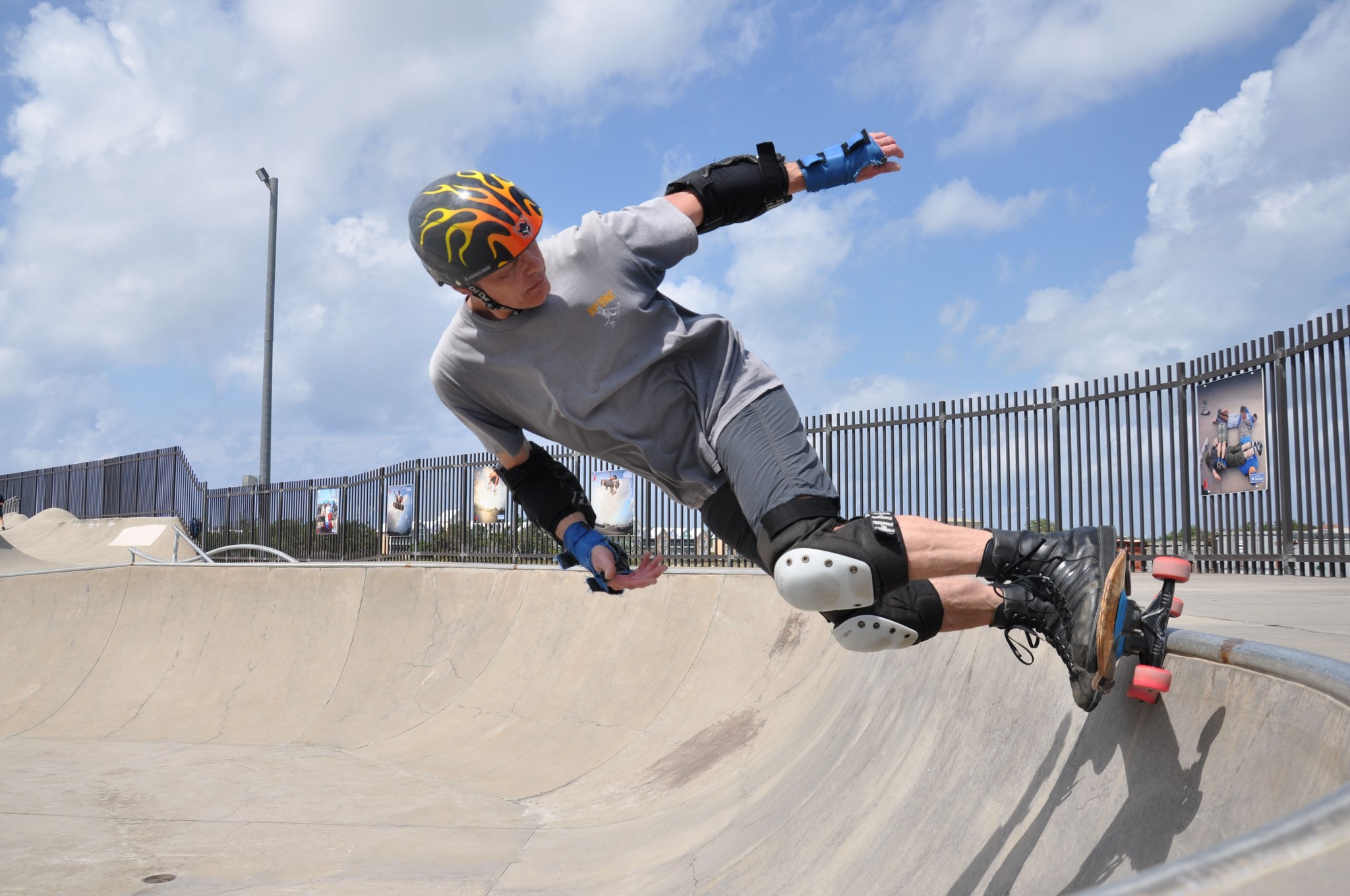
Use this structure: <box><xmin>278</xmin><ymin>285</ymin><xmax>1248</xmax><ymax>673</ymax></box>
<box><xmin>1217</xmin><ymin>458</ymin><xmax>1221</xmax><ymax>469</ymax></box>
<box><xmin>1216</xmin><ymin>413</ymin><xmax>1221</xmax><ymax>418</ymax></box>
<box><xmin>1241</xmin><ymin>406</ymin><xmax>1244</xmax><ymax>413</ymax></box>
<box><xmin>1243</xmin><ymin>406</ymin><xmax>1246</xmax><ymax>411</ymax></box>
<box><xmin>1218</xmin><ymin>409</ymin><xmax>1221</xmax><ymax>412</ymax></box>
<box><xmin>1258</xmin><ymin>443</ymin><xmax>1263</xmax><ymax>456</ymax></box>
<box><xmin>1221</xmin><ymin>459</ymin><xmax>1226</xmax><ymax>469</ymax></box>
<box><xmin>1257</xmin><ymin>441</ymin><xmax>1261</xmax><ymax>445</ymax></box>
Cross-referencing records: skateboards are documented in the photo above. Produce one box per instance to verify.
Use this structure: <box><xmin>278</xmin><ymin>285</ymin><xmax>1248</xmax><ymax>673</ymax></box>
<box><xmin>1090</xmin><ymin>547</ymin><xmax>1193</xmax><ymax>706</ymax></box>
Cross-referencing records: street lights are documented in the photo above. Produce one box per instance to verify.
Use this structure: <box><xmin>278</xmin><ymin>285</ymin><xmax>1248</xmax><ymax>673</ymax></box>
<box><xmin>253</xmin><ymin>166</ymin><xmax>281</xmax><ymax>562</ymax></box>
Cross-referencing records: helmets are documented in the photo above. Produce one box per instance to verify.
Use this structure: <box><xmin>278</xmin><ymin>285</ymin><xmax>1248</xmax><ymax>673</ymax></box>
<box><xmin>409</xmin><ymin>170</ymin><xmax>543</xmax><ymax>288</ymax></box>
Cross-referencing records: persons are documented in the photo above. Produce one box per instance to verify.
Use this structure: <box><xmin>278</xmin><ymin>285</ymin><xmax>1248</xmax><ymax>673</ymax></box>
<box><xmin>601</xmin><ymin>475</ymin><xmax>619</xmax><ymax>489</ymax></box>
<box><xmin>1205</xmin><ymin>406</ymin><xmax>1262</xmax><ymax>486</ymax></box>
<box><xmin>407</xmin><ymin>128</ymin><xmax>1116</xmax><ymax>713</ymax></box>
<box><xmin>0</xmin><ymin>493</ymin><xmax>5</xmax><ymax>530</ymax></box>
<box><xmin>321</xmin><ymin>501</ymin><xmax>332</xmax><ymax>532</ymax></box>
<box><xmin>488</xmin><ymin>466</ymin><xmax>499</xmax><ymax>492</ymax></box>
<box><xmin>394</xmin><ymin>490</ymin><xmax>403</xmax><ymax>504</ymax></box>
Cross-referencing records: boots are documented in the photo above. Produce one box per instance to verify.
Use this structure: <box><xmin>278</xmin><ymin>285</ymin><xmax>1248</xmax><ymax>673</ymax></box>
<box><xmin>990</xmin><ymin>583</ymin><xmax>1102</xmax><ymax>712</ymax></box>
<box><xmin>976</xmin><ymin>526</ymin><xmax>1117</xmax><ymax>673</ymax></box>
